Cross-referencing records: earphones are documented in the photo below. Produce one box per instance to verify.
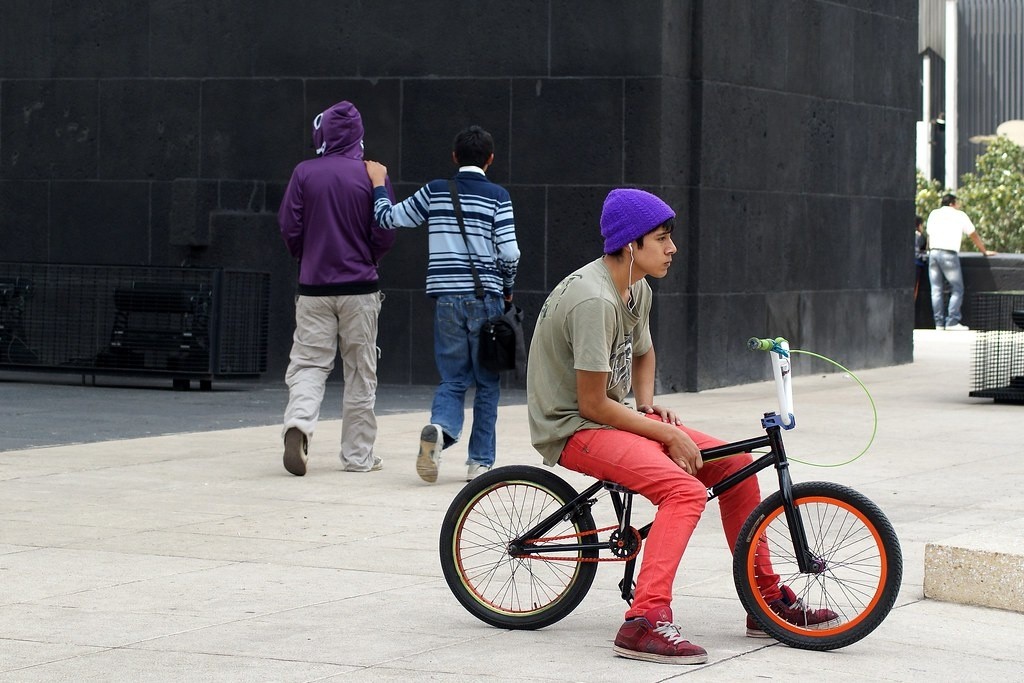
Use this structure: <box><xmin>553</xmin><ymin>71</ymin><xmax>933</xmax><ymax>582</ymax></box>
<box><xmin>628</xmin><ymin>242</ymin><xmax>633</xmax><ymax>250</ymax></box>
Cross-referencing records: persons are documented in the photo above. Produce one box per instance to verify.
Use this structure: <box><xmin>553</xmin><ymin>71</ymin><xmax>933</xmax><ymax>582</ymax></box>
<box><xmin>908</xmin><ymin>192</ymin><xmax>999</xmax><ymax>330</ymax></box>
<box><xmin>273</xmin><ymin>96</ymin><xmax>399</xmax><ymax>481</ymax></box>
<box><xmin>521</xmin><ymin>185</ymin><xmax>843</xmax><ymax>667</ymax></box>
<box><xmin>363</xmin><ymin>125</ymin><xmax>524</xmax><ymax>484</ymax></box>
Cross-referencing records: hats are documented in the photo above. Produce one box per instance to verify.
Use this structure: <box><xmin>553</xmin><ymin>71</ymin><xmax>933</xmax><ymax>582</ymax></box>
<box><xmin>600</xmin><ymin>188</ymin><xmax>675</xmax><ymax>254</ymax></box>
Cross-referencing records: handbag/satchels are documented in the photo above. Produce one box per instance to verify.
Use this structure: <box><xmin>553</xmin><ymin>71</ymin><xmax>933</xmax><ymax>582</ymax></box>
<box><xmin>479</xmin><ymin>298</ymin><xmax>529</xmax><ymax>380</ymax></box>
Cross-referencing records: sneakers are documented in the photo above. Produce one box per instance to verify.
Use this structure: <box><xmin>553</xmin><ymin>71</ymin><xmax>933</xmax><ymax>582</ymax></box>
<box><xmin>466</xmin><ymin>465</ymin><xmax>492</xmax><ymax>481</ymax></box>
<box><xmin>283</xmin><ymin>426</ymin><xmax>308</xmax><ymax>476</ymax></box>
<box><xmin>346</xmin><ymin>455</ymin><xmax>383</xmax><ymax>472</ymax></box>
<box><xmin>416</xmin><ymin>424</ymin><xmax>443</xmax><ymax>483</ymax></box>
<box><xmin>936</xmin><ymin>323</ymin><xmax>969</xmax><ymax>330</ymax></box>
<box><xmin>746</xmin><ymin>585</ymin><xmax>840</xmax><ymax>638</ymax></box>
<box><xmin>612</xmin><ymin>605</ymin><xmax>707</xmax><ymax>664</ymax></box>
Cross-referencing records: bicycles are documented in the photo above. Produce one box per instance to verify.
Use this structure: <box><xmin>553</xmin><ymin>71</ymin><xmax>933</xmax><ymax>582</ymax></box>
<box><xmin>437</xmin><ymin>336</ymin><xmax>903</xmax><ymax>651</ymax></box>
